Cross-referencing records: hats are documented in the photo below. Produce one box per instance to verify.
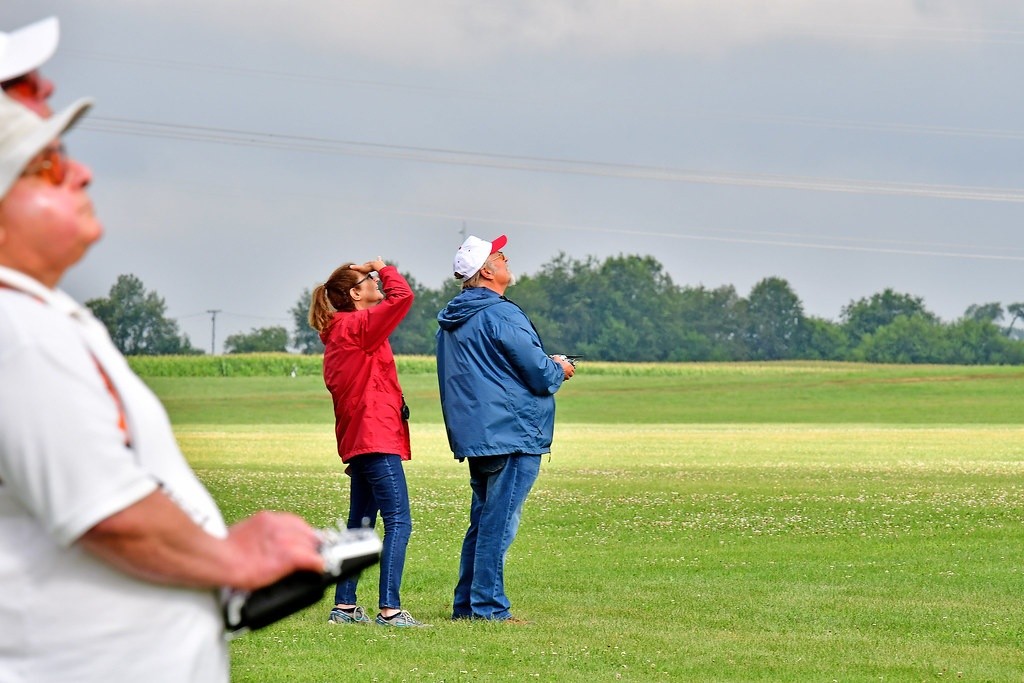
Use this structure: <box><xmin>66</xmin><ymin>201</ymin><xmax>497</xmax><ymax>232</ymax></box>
<box><xmin>0</xmin><ymin>16</ymin><xmax>59</xmax><ymax>83</ymax></box>
<box><xmin>453</xmin><ymin>235</ymin><xmax>507</xmax><ymax>282</ymax></box>
<box><xmin>0</xmin><ymin>88</ymin><xmax>92</xmax><ymax>198</ymax></box>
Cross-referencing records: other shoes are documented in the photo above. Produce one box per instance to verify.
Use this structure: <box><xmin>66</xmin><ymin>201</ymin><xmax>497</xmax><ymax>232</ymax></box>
<box><xmin>506</xmin><ymin>616</ymin><xmax>534</xmax><ymax>624</ymax></box>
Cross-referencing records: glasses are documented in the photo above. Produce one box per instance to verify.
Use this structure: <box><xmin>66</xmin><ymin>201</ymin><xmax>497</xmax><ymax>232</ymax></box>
<box><xmin>349</xmin><ymin>273</ymin><xmax>373</xmax><ymax>291</ymax></box>
<box><xmin>5</xmin><ymin>75</ymin><xmax>37</xmax><ymax>101</ymax></box>
<box><xmin>481</xmin><ymin>252</ymin><xmax>505</xmax><ymax>269</ymax></box>
<box><xmin>23</xmin><ymin>145</ymin><xmax>66</xmax><ymax>185</ymax></box>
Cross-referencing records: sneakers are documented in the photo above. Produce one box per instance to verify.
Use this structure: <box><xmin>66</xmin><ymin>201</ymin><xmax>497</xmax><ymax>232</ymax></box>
<box><xmin>375</xmin><ymin>610</ymin><xmax>433</xmax><ymax>628</ymax></box>
<box><xmin>328</xmin><ymin>606</ymin><xmax>372</xmax><ymax>625</ymax></box>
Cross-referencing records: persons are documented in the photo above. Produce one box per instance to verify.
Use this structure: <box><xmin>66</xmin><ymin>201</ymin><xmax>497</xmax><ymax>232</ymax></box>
<box><xmin>436</xmin><ymin>234</ymin><xmax>575</xmax><ymax>622</ymax></box>
<box><xmin>0</xmin><ymin>15</ymin><xmax>326</xmax><ymax>683</ymax></box>
<box><xmin>308</xmin><ymin>255</ymin><xmax>434</xmax><ymax>628</ymax></box>
